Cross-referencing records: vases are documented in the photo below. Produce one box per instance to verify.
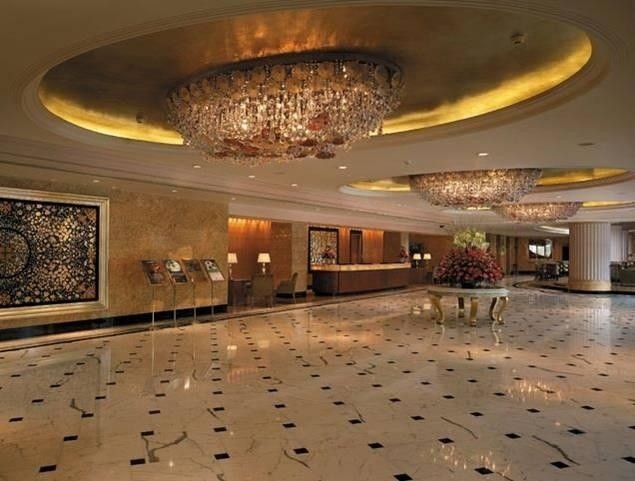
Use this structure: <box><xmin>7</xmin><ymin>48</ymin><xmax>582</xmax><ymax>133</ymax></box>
<box><xmin>460</xmin><ymin>282</ymin><xmax>478</xmax><ymax>289</ymax></box>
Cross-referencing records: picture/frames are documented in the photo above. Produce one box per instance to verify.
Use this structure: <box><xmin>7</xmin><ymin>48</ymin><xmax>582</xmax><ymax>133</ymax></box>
<box><xmin>308</xmin><ymin>226</ymin><xmax>340</xmax><ymax>273</ymax></box>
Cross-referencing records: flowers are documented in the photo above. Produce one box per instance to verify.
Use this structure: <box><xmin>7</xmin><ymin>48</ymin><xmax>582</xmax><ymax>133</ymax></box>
<box><xmin>432</xmin><ymin>245</ymin><xmax>505</xmax><ymax>288</ymax></box>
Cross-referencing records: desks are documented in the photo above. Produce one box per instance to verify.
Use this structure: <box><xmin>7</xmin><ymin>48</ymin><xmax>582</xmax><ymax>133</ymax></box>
<box><xmin>426</xmin><ymin>285</ymin><xmax>511</xmax><ymax>328</ymax></box>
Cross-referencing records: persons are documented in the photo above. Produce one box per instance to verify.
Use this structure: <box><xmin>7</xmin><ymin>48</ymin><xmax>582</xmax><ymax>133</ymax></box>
<box><xmin>399</xmin><ymin>245</ymin><xmax>408</xmax><ymax>263</ymax></box>
<box><xmin>321</xmin><ymin>241</ymin><xmax>337</xmax><ymax>265</ymax></box>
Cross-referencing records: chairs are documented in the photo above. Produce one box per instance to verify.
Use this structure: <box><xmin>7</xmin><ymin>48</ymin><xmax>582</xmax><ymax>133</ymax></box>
<box><xmin>228</xmin><ymin>272</ymin><xmax>299</xmax><ymax>310</ymax></box>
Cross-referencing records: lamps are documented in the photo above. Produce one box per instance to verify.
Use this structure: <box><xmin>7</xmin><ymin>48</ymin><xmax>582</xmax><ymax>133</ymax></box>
<box><xmin>413</xmin><ymin>253</ymin><xmax>433</xmax><ymax>273</ymax></box>
<box><xmin>257</xmin><ymin>252</ymin><xmax>271</xmax><ymax>277</ymax></box>
<box><xmin>227</xmin><ymin>252</ymin><xmax>238</xmax><ymax>280</ymax></box>
<box><xmin>409</xmin><ymin>169</ymin><xmax>543</xmax><ymax>210</ymax></box>
<box><xmin>491</xmin><ymin>202</ymin><xmax>584</xmax><ymax>223</ymax></box>
<box><xmin>169</xmin><ymin>52</ymin><xmax>402</xmax><ymax>167</ymax></box>
